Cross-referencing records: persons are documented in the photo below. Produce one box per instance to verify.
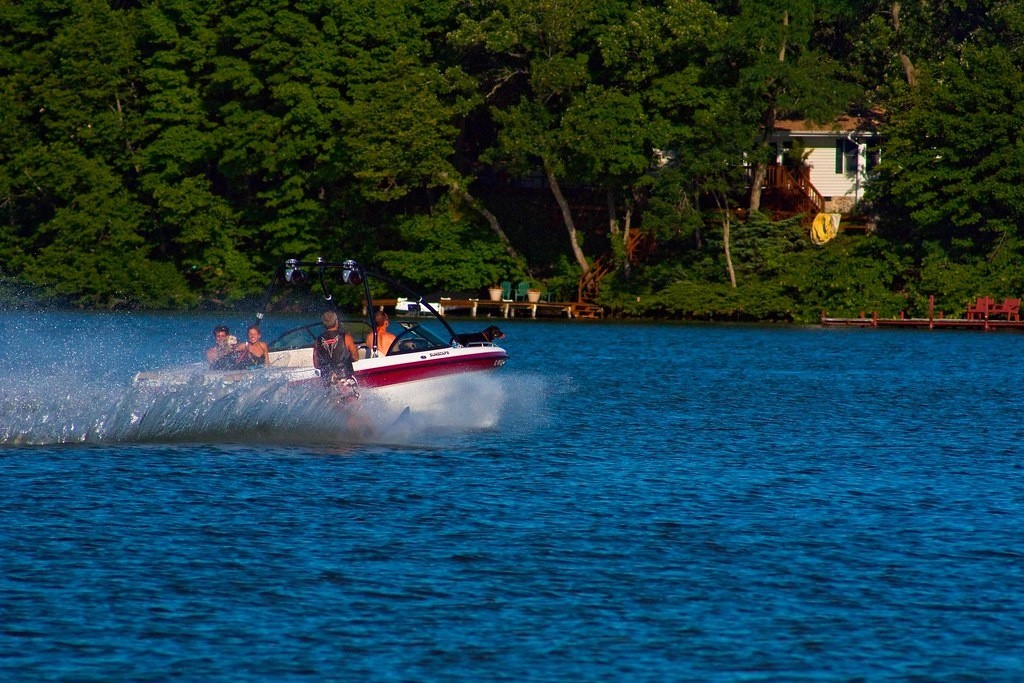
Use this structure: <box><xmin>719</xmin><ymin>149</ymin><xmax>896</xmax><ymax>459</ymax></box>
<box><xmin>205</xmin><ymin>324</ymin><xmax>246</xmax><ymax>363</ymax></box>
<box><xmin>312</xmin><ymin>311</ymin><xmax>380</xmax><ymax>441</ymax></box>
<box><xmin>230</xmin><ymin>326</ymin><xmax>270</xmax><ymax>370</ymax></box>
<box><xmin>363</xmin><ymin>309</ymin><xmax>401</xmax><ymax>357</ymax></box>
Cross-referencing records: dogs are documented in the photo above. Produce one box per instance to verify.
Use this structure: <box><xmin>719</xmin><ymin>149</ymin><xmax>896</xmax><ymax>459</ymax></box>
<box><xmin>449</xmin><ymin>325</ymin><xmax>506</xmax><ymax>347</ymax></box>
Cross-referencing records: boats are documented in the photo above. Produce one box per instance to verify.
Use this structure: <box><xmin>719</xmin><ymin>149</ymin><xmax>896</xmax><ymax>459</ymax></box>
<box><xmin>133</xmin><ymin>259</ymin><xmax>511</xmax><ymax>423</ymax></box>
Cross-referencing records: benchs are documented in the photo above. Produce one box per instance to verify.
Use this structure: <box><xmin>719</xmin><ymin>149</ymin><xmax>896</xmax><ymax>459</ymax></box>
<box><xmin>967</xmin><ymin>296</ymin><xmax>1022</xmax><ymax>320</ymax></box>
